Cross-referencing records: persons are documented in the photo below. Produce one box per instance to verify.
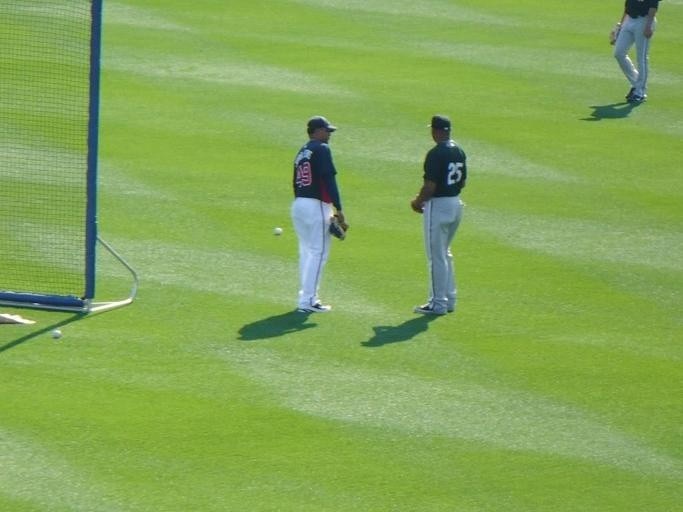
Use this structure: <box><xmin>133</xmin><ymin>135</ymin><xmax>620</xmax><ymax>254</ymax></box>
<box><xmin>288</xmin><ymin>116</ymin><xmax>346</xmax><ymax>315</ymax></box>
<box><xmin>410</xmin><ymin>114</ymin><xmax>467</xmax><ymax>317</ymax></box>
<box><xmin>611</xmin><ymin>1</ymin><xmax>660</xmax><ymax>104</ymax></box>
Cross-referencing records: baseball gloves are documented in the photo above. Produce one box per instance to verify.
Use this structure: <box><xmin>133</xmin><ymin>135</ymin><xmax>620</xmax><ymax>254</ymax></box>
<box><xmin>329</xmin><ymin>214</ymin><xmax>349</xmax><ymax>240</ymax></box>
<box><xmin>608</xmin><ymin>23</ymin><xmax>621</xmax><ymax>44</ymax></box>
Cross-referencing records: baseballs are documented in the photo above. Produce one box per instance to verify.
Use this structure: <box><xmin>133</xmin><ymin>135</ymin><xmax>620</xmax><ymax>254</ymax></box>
<box><xmin>275</xmin><ymin>228</ymin><xmax>283</xmax><ymax>235</ymax></box>
<box><xmin>53</xmin><ymin>331</ymin><xmax>61</xmax><ymax>337</ymax></box>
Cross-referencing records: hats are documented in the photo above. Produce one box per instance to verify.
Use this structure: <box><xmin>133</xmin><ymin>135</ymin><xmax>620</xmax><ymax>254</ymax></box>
<box><xmin>308</xmin><ymin>116</ymin><xmax>336</xmax><ymax>134</ymax></box>
<box><xmin>428</xmin><ymin>116</ymin><xmax>450</xmax><ymax>130</ymax></box>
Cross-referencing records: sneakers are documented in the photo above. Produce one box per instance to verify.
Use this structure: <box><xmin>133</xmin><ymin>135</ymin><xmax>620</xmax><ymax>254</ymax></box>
<box><xmin>628</xmin><ymin>94</ymin><xmax>647</xmax><ymax>103</ymax></box>
<box><xmin>297</xmin><ymin>302</ymin><xmax>331</xmax><ymax>313</ymax></box>
<box><xmin>415</xmin><ymin>303</ymin><xmax>434</xmax><ymax>314</ymax></box>
